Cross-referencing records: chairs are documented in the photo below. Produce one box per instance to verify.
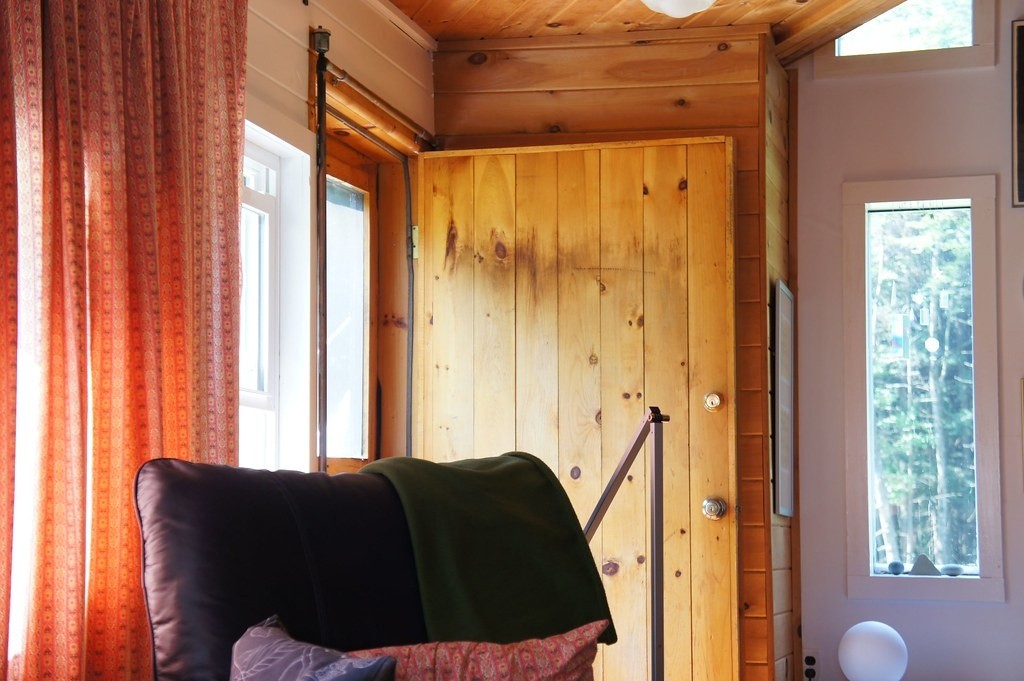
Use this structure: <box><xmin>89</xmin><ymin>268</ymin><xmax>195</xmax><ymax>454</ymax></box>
<box><xmin>134</xmin><ymin>453</ymin><xmax>617</xmax><ymax>681</ymax></box>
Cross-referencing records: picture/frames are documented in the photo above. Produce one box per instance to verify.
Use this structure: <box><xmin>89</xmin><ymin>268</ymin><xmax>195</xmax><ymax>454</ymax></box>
<box><xmin>772</xmin><ymin>279</ymin><xmax>795</xmax><ymax>519</ymax></box>
<box><xmin>1010</xmin><ymin>18</ymin><xmax>1024</xmax><ymax>207</ymax></box>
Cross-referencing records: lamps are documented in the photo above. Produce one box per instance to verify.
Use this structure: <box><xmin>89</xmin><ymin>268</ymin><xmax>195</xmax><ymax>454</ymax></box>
<box><xmin>643</xmin><ymin>0</ymin><xmax>716</xmax><ymax>17</ymax></box>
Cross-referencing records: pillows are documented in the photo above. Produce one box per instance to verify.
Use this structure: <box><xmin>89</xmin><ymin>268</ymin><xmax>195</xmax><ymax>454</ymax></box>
<box><xmin>227</xmin><ymin>613</ymin><xmax>394</xmax><ymax>681</ymax></box>
<box><xmin>345</xmin><ymin>621</ymin><xmax>609</xmax><ymax>681</ymax></box>
